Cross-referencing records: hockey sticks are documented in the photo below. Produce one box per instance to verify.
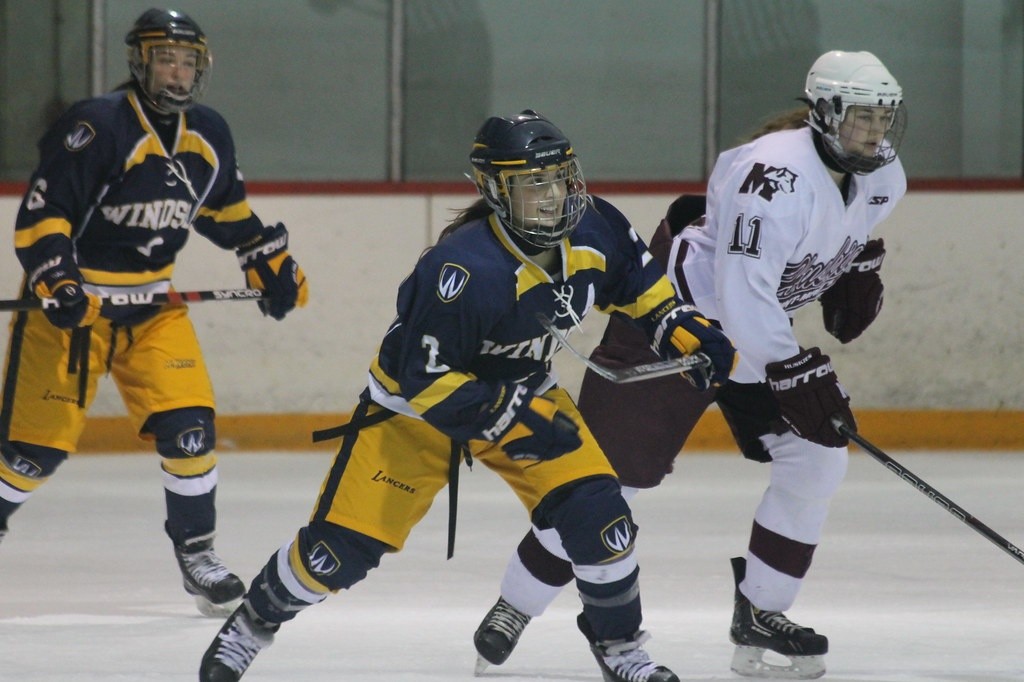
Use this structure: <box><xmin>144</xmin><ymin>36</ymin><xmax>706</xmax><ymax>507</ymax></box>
<box><xmin>0</xmin><ymin>286</ymin><xmax>271</xmax><ymax>315</ymax></box>
<box><xmin>829</xmin><ymin>416</ymin><xmax>1024</xmax><ymax>567</ymax></box>
<box><xmin>523</xmin><ymin>299</ymin><xmax>712</xmax><ymax>388</ymax></box>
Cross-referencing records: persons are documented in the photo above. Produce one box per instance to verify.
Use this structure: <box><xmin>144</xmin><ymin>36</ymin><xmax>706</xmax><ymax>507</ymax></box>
<box><xmin>199</xmin><ymin>110</ymin><xmax>737</xmax><ymax>682</ymax></box>
<box><xmin>473</xmin><ymin>52</ymin><xmax>908</xmax><ymax>665</ymax></box>
<box><xmin>0</xmin><ymin>7</ymin><xmax>309</xmax><ymax>607</ymax></box>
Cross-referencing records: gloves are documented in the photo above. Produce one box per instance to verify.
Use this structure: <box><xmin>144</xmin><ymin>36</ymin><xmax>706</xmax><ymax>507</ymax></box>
<box><xmin>820</xmin><ymin>238</ymin><xmax>886</xmax><ymax>343</ymax></box>
<box><xmin>764</xmin><ymin>345</ymin><xmax>858</xmax><ymax>448</ymax></box>
<box><xmin>476</xmin><ymin>378</ymin><xmax>583</xmax><ymax>460</ymax></box>
<box><xmin>648</xmin><ymin>299</ymin><xmax>739</xmax><ymax>390</ymax></box>
<box><xmin>231</xmin><ymin>221</ymin><xmax>308</xmax><ymax>320</ymax></box>
<box><xmin>29</xmin><ymin>254</ymin><xmax>102</xmax><ymax>330</ymax></box>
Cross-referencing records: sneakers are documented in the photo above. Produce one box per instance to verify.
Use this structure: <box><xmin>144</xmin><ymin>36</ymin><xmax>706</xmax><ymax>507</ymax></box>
<box><xmin>729</xmin><ymin>556</ymin><xmax>828</xmax><ymax>679</ymax></box>
<box><xmin>198</xmin><ymin>593</ymin><xmax>281</xmax><ymax>682</ymax></box>
<box><xmin>164</xmin><ymin>519</ymin><xmax>246</xmax><ymax>617</ymax></box>
<box><xmin>576</xmin><ymin>611</ymin><xmax>681</xmax><ymax>682</ymax></box>
<box><xmin>472</xmin><ymin>595</ymin><xmax>532</xmax><ymax>676</ymax></box>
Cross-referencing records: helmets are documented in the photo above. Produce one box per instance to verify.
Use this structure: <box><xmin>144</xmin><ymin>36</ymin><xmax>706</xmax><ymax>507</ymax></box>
<box><xmin>469</xmin><ymin>109</ymin><xmax>587</xmax><ymax>248</ymax></box>
<box><xmin>126</xmin><ymin>7</ymin><xmax>212</xmax><ymax>111</ymax></box>
<box><xmin>799</xmin><ymin>50</ymin><xmax>908</xmax><ymax>176</ymax></box>
